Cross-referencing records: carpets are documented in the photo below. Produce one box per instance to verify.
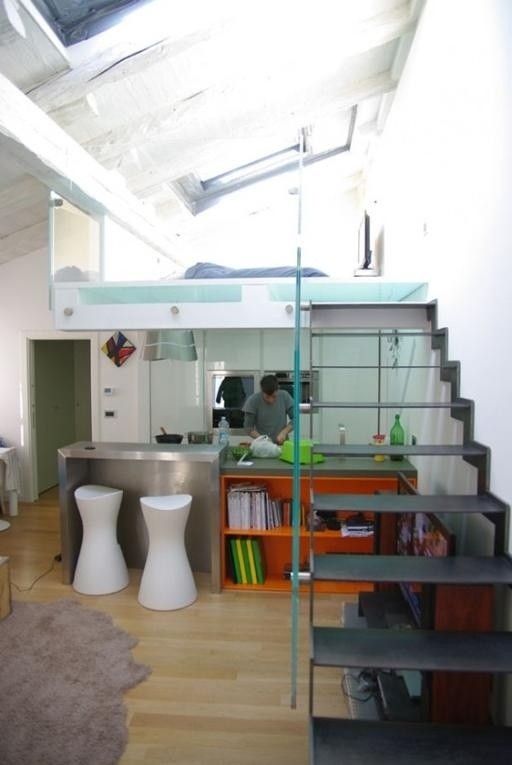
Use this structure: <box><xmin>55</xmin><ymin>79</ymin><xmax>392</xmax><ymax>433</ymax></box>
<box><xmin>0</xmin><ymin>598</ymin><xmax>154</xmax><ymax>765</ymax></box>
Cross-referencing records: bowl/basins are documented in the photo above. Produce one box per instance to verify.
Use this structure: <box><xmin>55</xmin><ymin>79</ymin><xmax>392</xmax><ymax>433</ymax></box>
<box><xmin>155</xmin><ymin>435</ymin><xmax>183</xmax><ymax>443</ymax></box>
<box><xmin>229</xmin><ymin>447</ymin><xmax>252</xmax><ymax>461</ymax></box>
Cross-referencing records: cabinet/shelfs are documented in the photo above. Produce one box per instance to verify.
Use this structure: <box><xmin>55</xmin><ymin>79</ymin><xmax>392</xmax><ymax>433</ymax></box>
<box><xmin>340</xmin><ymin>590</ymin><xmax>429</xmax><ymax>722</ymax></box>
<box><xmin>218</xmin><ymin>474</ymin><xmax>415</xmax><ymax>594</ymax></box>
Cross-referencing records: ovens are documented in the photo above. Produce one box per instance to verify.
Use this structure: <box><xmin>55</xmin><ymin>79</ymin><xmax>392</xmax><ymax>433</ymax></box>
<box><xmin>264</xmin><ymin>370</ymin><xmax>312</xmax><ymax>413</ymax></box>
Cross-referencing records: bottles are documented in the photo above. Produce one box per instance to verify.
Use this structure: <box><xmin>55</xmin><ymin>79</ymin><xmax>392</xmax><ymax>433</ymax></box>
<box><xmin>218</xmin><ymin>417</ymin><xmax>230</xmax><ymax>444</ymax></box>
<box><xmin>374</xmin><ymin>414</ymin><xmax>404</xmax><ymax>461</ymax></box>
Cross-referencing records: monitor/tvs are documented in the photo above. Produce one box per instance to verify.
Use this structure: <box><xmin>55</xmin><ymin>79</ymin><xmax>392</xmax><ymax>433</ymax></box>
<box><xmin>357</xmin><ymin>210</ymin><xmax>372</xmax><ymax>269</ymax></box>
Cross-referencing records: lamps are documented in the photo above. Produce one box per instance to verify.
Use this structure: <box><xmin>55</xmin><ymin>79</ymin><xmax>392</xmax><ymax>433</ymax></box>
<box><xmin>144</xmin><ymin>329</ymin><xmax>198</xmax><ymax>362</ymax></box>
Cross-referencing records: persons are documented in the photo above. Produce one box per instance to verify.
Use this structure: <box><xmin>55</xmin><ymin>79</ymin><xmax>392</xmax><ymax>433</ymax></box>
<box><xmin>241</xmin><ymin>375</ymin><xmax>294</xmax><ymax>445</ymax></box>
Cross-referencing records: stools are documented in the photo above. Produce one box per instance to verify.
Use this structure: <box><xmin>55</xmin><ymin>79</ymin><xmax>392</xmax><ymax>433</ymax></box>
<box><xmin>137</xmin><ymin>493</ymin><xmax>198</xmax><ymax>611</ymax></box>
<box><xmin>72</xmin><ymin>484</ymin><xmax>129</xmax><ymax>596</ymax></box>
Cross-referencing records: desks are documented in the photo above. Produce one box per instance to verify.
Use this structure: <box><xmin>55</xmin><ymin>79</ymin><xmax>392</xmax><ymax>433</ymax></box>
<box><xmin>57</xmin><ymin>440</ymin><xmax>228</xmax><ymax>593</ymax></box>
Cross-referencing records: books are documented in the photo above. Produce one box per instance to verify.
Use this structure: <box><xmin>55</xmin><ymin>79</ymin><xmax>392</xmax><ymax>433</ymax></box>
<box><xmin>341</xmin><ymin>518</ymin><xmax>375</xmax><ymax>538</ymax></box>
<box><xmin>225</xmin><ymin>536</ymin><xmax>264</xmax><ymax>584</ymax></box>
<box><xmin>227</xmin><ymin>485</ymin><xmax>306</xmax><ymax>529</ymax></box>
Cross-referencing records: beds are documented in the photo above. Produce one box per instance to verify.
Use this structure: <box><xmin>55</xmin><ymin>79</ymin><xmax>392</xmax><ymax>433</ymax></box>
<box><xmin>52</xmin><ymin>276</ymin><xmax>430</xmax><ymax>330</ymax></box>
